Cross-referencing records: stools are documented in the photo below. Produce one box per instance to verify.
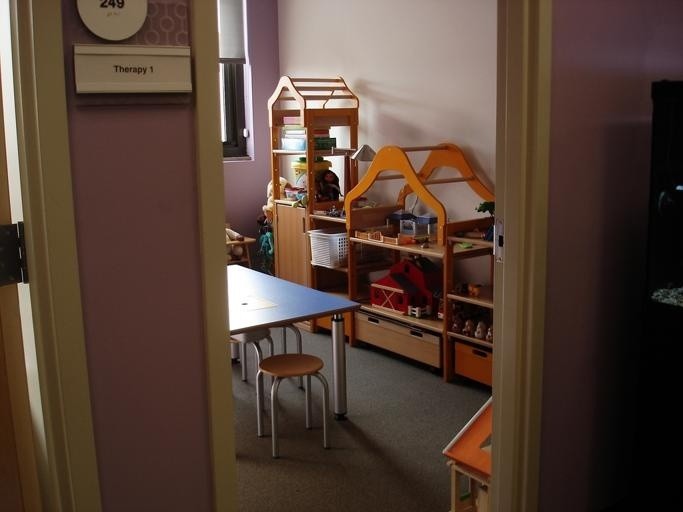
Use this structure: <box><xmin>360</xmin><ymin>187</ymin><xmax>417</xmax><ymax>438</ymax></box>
<box><xmin>241</xmin><ymin>323</ymin><xmax>304</xmax><ymax>391</ymax></box>
<box><xmin>256</xmin><ymin>353</ymin><xmax>331</xmax><ymax>459</ymax></box>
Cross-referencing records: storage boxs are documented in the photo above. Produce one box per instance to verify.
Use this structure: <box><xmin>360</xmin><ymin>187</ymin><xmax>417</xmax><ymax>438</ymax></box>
<box><xmin>305</xmin><ymin>225</ymin><xmax>348</xmax><ymax>268</ymax></box>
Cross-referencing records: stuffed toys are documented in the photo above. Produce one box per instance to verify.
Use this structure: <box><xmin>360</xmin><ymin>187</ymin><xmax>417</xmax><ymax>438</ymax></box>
<box><xmin>262</xmin><ymin>176</ymin><xmax>293</xmax><ymax>224</ymax></box>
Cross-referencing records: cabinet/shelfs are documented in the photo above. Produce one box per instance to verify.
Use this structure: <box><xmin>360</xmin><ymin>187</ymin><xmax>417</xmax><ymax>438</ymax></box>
<box><xmin>266</xmin><ymin>77</ymin><xmax>356</xmax><ymax>344</ymax></box>
<box><xmin>346</xmin><ymin>143</ymin><xmax>496</xmax><ymax>391</ymax></box>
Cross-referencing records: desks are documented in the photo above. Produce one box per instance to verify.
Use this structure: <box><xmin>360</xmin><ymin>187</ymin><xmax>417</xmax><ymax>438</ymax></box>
<box><xmin>226</xmin><ymin>264</ymin><xmax>361</xmax><ymax>422</ymax></box>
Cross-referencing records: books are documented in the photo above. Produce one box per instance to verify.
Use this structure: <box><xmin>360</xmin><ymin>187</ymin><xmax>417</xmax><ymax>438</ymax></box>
<box><xmin>280</xmin><ymin>116</ymin><xmax>337</xmax><ymax>150</ymax></box>
